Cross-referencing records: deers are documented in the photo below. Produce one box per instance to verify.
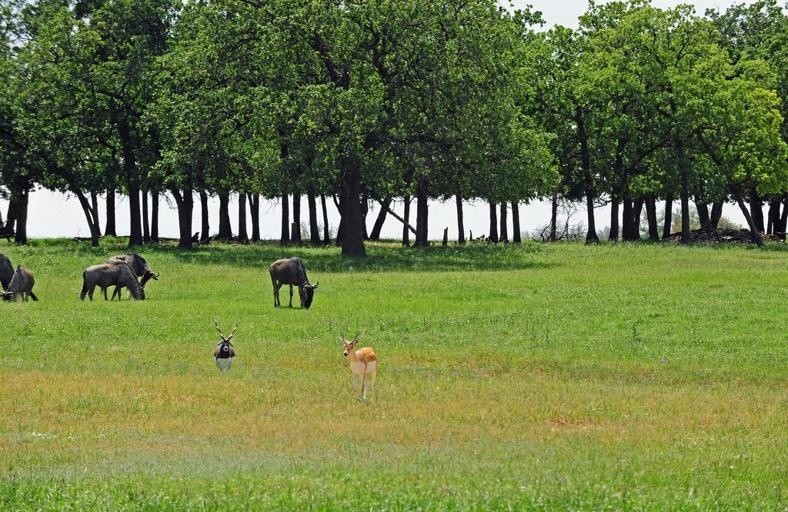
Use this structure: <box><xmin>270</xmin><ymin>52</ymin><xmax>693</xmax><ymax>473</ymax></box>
<box><xmin>337</xmin><ymin>330</ymin><xmax>378</xmax><ymax>400</ymax></box>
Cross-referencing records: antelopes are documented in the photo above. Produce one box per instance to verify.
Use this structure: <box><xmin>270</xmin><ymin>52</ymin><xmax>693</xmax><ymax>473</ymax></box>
<box><xmin>212</xmin><ymin>318</ymin><xmax>241</xmax><ymax>375</ymax></box>
